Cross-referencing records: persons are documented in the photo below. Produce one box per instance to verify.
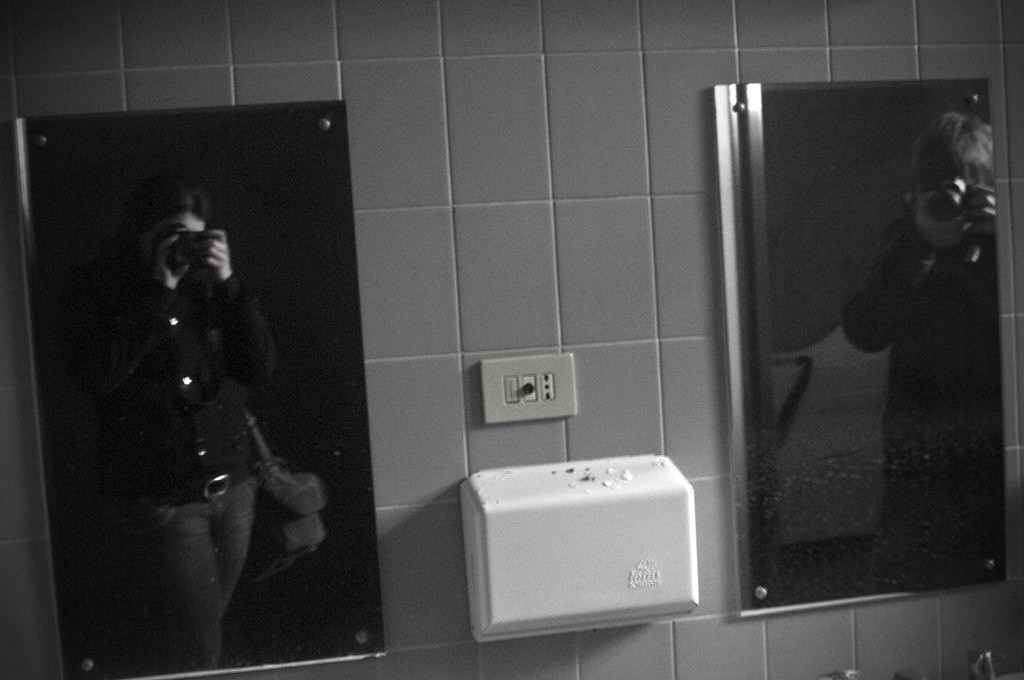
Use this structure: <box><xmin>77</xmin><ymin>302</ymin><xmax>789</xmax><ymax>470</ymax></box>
<box><xmin>841</xmin><ymin>113</ymin><xmax>1006</xmax><ymax>592</ymax></box>
<box><xmin>57</xmin><ymin>171</ymin><xmax>282</xmax><ymax>672</ymax></box>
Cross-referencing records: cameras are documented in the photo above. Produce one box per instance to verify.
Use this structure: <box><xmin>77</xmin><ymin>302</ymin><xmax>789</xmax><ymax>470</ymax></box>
<box><xmin>913</xmin><ymin>176</ymin><xmax>979</xmax><ymax>221</ymax></box>
<box><xmin>167</xmin><ymin>230</ymin><xmax>216</xmax><ymax>268</ymax></box>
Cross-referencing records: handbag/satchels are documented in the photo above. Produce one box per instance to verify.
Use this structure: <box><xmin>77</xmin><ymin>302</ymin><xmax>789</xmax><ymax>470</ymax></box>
<box><xmin>256</xmin><ymin>458</ymin><xmax>326</xmax><ymax>551</ymax></box>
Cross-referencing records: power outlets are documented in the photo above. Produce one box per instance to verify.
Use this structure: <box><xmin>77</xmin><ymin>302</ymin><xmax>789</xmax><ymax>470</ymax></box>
<box><xmin>478</xmin><ymin>351</ymin><xmax>579</xmax><ymax>425</ymax></box>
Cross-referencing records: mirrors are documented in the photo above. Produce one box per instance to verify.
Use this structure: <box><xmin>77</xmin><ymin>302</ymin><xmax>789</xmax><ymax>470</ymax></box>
<box><xmin>12</xmin><ymin>100</ymin><xmax>387</xmax><ymax>680</ymax></box>
<box><xmin>712</xmin><ymin>74</ymin><xmax>1009</xmax><ymax>622</ymax></box>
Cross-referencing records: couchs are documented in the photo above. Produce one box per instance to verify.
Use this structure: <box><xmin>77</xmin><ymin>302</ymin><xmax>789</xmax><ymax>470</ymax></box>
<box><xmin>759</xmin><ymin>323</ymin><xmax>889</xmax><ymax>550</ymax></box>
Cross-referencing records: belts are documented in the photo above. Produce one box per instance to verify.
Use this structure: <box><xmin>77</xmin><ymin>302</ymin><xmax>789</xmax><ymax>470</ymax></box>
<box><xmin>155</xmin><ymin>470</ymin><xmax>246</xmax><ymax>507</ymax></box>
